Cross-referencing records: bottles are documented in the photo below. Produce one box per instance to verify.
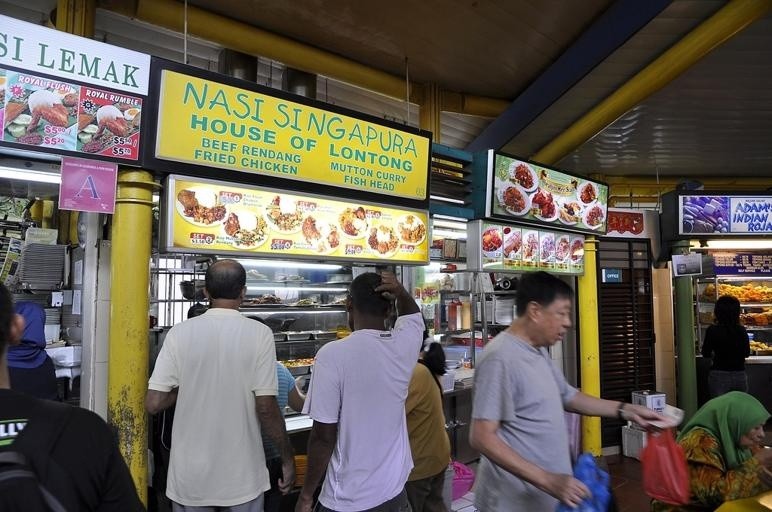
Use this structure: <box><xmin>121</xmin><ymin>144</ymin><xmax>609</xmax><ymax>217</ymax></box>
<box><xmin>449</xmin><ymin>298</ymin><xmax>477</xmax><ymax>331</ymax></box>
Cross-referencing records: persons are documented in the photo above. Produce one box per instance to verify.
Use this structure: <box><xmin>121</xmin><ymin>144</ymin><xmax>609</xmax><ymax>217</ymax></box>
<box><xmin>464</xmin><ymin>272</ymin><xmax>666</xmax><ymax>512</ymax></box>
<box><xmin>8</xmin><ymin>300</ymin><xmax>59</xmax><ymax>400</ymax></box>
<box><xmin>387</xmin><ymin>313</ymin><xmax>451</xmax><ymax>512</ymax></box>
<box><xmin>700</xmin><ymin>294</ymin><xmax>751</xmax><ymax>398</ymax></box>
<box><xmin>0</xmin><ymin>281</ymin><xmax>149</xmax><ymax>511</ymax></box>
<box><xmin>649</xmin><ymin>390</ymin><xmax>772</xmax><ymax>512</ymax></box>
<box><xmin>143</xmin><ymin>258</ymin><xmax>298</xmax><ymax>512</ymax></box>
<box><xmin>295</xmin><ymin>270</ymin><xmax>427</xmax><ymax>512</ymax></box>
<box><xmin>244</xmin><ymin>314</ymin><xmax>305</xmax><ymax>512</ymax></box>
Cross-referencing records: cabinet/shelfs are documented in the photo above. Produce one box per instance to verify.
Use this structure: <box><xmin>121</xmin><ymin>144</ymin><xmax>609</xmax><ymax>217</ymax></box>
<box><xmin>183</xmin><ymin>259</ymin><xmax>404</xmax><ymax>417</ymax></box>
<box><xmin>437</xmin><ymin>269</ymin><xmax>518</xmax><ymax>370</ymax></box>
<box><xmin>696</xmin><ymin>276</ymin><xmax>772</xmax><ymax>356</ymax></box>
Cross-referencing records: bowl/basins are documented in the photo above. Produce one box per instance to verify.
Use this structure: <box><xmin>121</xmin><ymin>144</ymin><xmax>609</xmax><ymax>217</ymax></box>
<box><xmin>180</xmin><ymin>281</ymin><xmax>207</xmax><ymax>299</ymax></box>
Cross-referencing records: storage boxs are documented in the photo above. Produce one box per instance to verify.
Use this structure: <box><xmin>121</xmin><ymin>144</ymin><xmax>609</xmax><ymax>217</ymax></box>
<box><xmin>622</xmin><ymin>389</ymin><xmax>666</xmax><ymax>460</ymax></box>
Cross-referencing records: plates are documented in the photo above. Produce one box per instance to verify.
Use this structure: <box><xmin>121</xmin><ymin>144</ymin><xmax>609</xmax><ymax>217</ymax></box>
<box><xmin>482</xmin><ymin>161</ymin><xmax>606</xmax><ymax>267</ymax></box>
<box><xmin>177</xmin><ymin>186</ymin><xmax>427</xmax><ymax>259</ymax></box>
<box><xmin>244</xmin><ymin>278</ymin><xmax>352</xmax><ymax>308</ymax></box>
<box><xmin>11</xmin><ymin>244</ymin><xmax>63</xmax><ymax>324</ymax></box>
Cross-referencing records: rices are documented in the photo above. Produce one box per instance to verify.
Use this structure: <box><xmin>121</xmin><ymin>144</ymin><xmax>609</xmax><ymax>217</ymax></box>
<box><xmin>187</xmin><ymin>185</ymin><xmax>218</xmax><ymax>210</ymax></box>
<box><xmin>235</xmin><ymin>209</ymin><xmax>257</xmax><ymax>230</ymax></box>
<box><xmin>376</xmin><ymin>226</ymin><xmax>392</xmax><ymax>242</ymax></box>
<box><xmin>96</xmin><ymin>105</ymin><xmax>124</xmax><ymax>125</ymax></box>
<box><xmin>28</xmin><ymin>88</ymin><xmax>64</xmax><ymax>113</ymax></box>
<box><xmin>353</xmin><ymin>217</ymin><xmax>366</xmax><ymax>232</ymax></box>
<box><xmin>122</xmin><ymin>109</ymin><xmax>140</xmax><ymax>121</ymax></box>
<box><xmin>279</xmin><ymin>194</ymin><xmax>297</xmax><ymax>214</ymax></box>
<box><xmin>403</xmin><ymin>215</ymin><xmax>419</xmax><ymax>231</ymax></box>
<box><xmin>314</xmin><ymin>218</ymin><xmax>330</xmax><ymax>239</ymax></box>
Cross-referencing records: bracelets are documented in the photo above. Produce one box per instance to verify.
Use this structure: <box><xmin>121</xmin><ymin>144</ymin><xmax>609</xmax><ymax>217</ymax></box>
<box><xmin>616</xmin><ymin>400</ymin><xmax>625</xmax><ymax>421</ymax></box>
